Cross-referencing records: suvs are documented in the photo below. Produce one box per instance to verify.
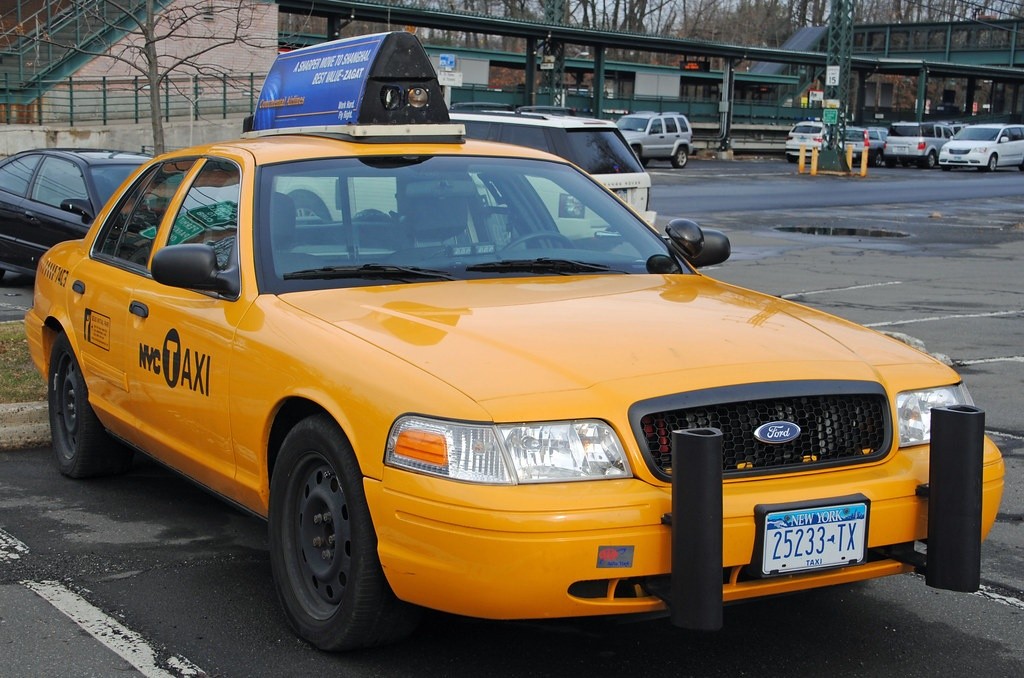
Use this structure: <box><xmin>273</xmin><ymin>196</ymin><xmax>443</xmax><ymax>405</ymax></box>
<box><xmin>274</xmin><ymin>102</ymin><xmax>657</xmax><ymax>252</ymax></box>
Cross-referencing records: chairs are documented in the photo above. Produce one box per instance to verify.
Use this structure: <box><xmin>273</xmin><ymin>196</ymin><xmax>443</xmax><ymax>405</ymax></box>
<box><xmin>387</xmin><ymin>171</ymin><xmax>469</xmax><ymax>264</ymax></box>
<box><xmin>268</xmin><ymin>190</ymin><xmax>322</xmax><ymax>277</ymax></box>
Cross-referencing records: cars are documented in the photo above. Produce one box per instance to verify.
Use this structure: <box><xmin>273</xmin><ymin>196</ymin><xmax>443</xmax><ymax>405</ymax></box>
<box><xmin>784</xmin><ymin>116</ymin><xmax>846</xmax><ymax>163</ymax></box>
<box><xmin>882</xmin><ymin>122</ymin><xmax>956</xmax><ymax>170</ymax></box>
<box><xmin>946</xmin><ymin>124</ymin><xmax>968</xmax><ymax>136</ymax></box>
<box><xmin>838</xmin><ymin>125</ymin><xmax>888</xmax><ymax>167</ymax></box>
<box><xmin>23</xmin><ymin>133</ymin><xmax>1005</xmax><ymax>656</ymax></box>
<box><xmin>0</xmin><ymin>148</ymin><xmax>159</xmax><ymax>283</ymax></box>
<box><xmin>614</xmin><ymin>114</ymin><xmax>693</xmax><ymax>168</ymax></box>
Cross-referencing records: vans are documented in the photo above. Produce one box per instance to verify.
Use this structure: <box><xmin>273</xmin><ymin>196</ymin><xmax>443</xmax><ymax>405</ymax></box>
<box><xmin>938</xmin><ymin>123</ymin><xmax>1024</xmax><ymax>171</ymax></box>
